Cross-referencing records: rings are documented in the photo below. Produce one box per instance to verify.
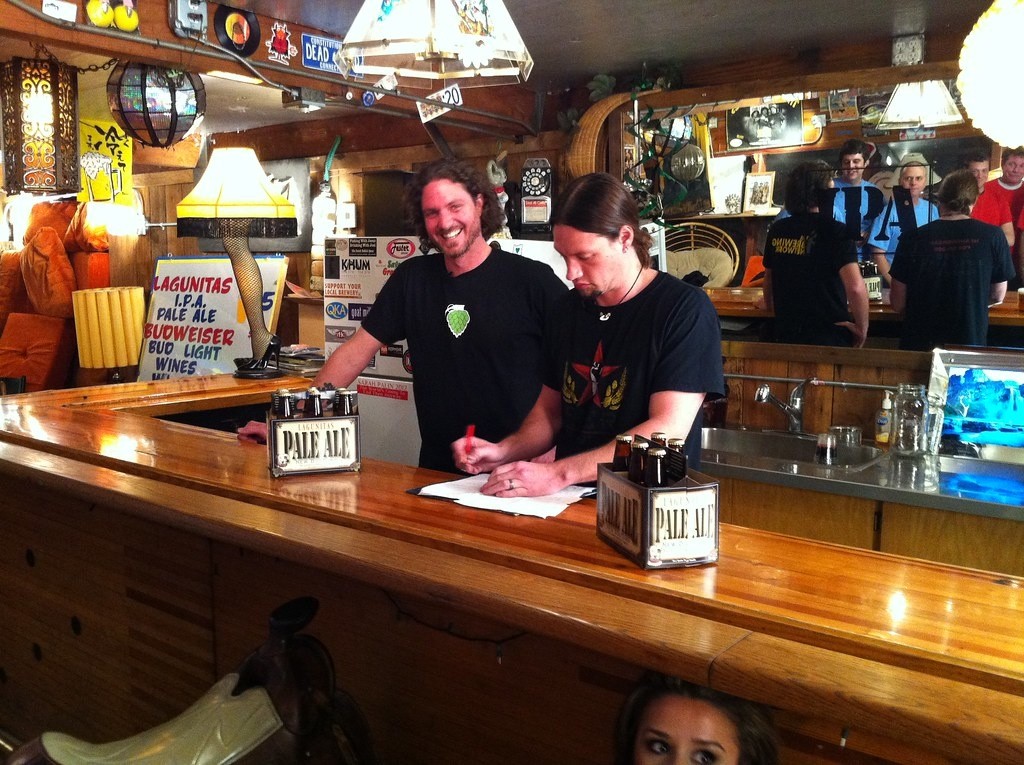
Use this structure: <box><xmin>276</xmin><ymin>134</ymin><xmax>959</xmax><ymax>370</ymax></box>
<box><xmin>509</xmin><ymin>479</ymin><xmax>514</xmax><ymax>490</ymax></box>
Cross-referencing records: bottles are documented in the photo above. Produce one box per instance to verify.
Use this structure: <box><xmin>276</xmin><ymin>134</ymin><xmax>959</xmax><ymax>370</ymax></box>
<box><xmin>274</xmin><ymin>387</ymin><xmax>353</xmax><ymax>419</ymax></box>
<box><xmin>614</xmin><ymin>430</ymin><xmax>686</xmax><ymax>486</ymax></box>
<box><xmin>889</xmin><ymin>382</ymin><xmax>929</xmax><ymax>459</ymax></box>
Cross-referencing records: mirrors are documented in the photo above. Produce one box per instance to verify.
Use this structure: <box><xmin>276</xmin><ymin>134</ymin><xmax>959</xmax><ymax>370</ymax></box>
<box><xmin>604</xmin><ymin>65</ymin><xmax>1024</xmax><ymax>372</ymax></box>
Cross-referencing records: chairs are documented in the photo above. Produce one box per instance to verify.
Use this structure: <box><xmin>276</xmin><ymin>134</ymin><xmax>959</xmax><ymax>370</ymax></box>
<box><xmin>665</xmin><ymin>222</ymin><xmax>740</xmax><ymax>288</ymax></box>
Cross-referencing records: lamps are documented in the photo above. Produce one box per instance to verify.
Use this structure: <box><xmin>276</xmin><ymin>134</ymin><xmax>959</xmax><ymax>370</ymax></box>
<box><xmin>174</xmin><ymin>146</ymin><xmax>297</xmax><ymax>381</ymax></box>
<box><xmin>877</xmin><ymin>75</ymin><xmax>963</xmax><ymax>130</ymax></box>
<box><xmin>334</xmin><ymin>1</ymin><xmax>535</xmax><ymax>94</ymax></box>
<box><xmin>72</xmin><ymin>283</ymin><xmax>147</xmax><ymax>383</ymax></box>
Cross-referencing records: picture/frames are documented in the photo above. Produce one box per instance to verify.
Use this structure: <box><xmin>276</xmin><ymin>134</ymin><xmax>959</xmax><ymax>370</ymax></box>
<box><xmin>743</xmin><ymin>170</ymin><xmax>776</xmax><ymax>212</ymax></box>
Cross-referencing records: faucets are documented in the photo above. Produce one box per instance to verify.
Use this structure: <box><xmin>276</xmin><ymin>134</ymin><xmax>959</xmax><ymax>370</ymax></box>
<box><xmin>752</xmin><ymin>375</ymin><xmax>821</xmax><ymax>434</ymax></box>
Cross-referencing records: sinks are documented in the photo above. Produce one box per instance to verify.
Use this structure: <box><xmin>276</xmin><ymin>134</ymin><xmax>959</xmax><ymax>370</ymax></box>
<box><xmin>697</xmin><ymin>425</ymin><xmax>880</xmax><ymax>471</ymax></box>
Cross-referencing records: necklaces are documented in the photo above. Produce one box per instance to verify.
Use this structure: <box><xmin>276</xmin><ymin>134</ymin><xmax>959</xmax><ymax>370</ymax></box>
<box><xmin>594</xmin><ymin>265</ymin><xmax>644</xmax><ymax>321</ymax></box>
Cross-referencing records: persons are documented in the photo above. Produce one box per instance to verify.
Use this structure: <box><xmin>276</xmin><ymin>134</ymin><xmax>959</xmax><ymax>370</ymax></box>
<box><xmin>964</xmin><ymin>145</ymin><xmax>1024</xmax><ymax>291</ymax></box>
<box><xmin>451</xmin><ymin>173</ymin><xmax>725</xmax><ymax>498</ymax></box>
<box><xmin>888</xmin><ymin>170</ymin><xmax>1016</xmax><ymax>351</ymax></box>
<box><xmin>744</xmin><ymin>106</ymin><xmax>786</xmax><ymax>137</ymax></box>
<box><xmin>614</xmin><ymin>669</ymin><xmax>779</xmax><ymax>765</ymax></box>
<box><xmin>750</xmin><ymin>181</ymin><xmax>769</xmax><ymax>204</ymax></box>
<box><xmin>773</xmin><ymin>138</ymin><xmax>886</xmax><ymax>273</ymax></box>
<box><xmin>868</xmin><ymin>161</ymin><xmax>939</xmax><ymax>288</ymax></box>
<box><xmin>238</xmin><ymin>161</ymin><xmax>570</xmax><ymax>477</ymax></box>
<box><xmin>753</xmin><ymin>160</ymin><xmax>868</xmax><ymax>348</ymax></box>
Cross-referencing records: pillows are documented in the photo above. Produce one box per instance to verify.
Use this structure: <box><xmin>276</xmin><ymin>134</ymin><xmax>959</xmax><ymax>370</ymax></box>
<box><xmin>24</xmin><ymin>201</ymin><xmax>78</xmax><ymax>252</ymax></box>
<box><xmin>20</xmin><ymin>227</ymin><xmax>75</xmax><ymax>317</ymax></box>
<box><xmin>64</xmin><ymin>202</ymin><xmax>109</xmax><ymax>250</ymax></box>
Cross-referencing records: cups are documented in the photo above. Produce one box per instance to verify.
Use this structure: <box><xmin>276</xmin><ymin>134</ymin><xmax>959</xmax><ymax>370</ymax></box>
<box><xmin>813</xmin><ymin>433</ymin><xmax>837</xmax><ymax>466</ymax></box>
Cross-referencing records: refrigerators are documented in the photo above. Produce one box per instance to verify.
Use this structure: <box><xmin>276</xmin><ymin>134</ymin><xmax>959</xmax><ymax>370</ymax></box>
<box><xmin>322</xmin><ymin>235</ymin><xmax>573</xmax><ymax>466</ymax></box>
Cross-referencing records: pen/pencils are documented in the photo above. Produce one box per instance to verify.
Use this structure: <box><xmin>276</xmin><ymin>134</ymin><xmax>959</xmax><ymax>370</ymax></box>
<box><xmin>465</xmin><ymin>424</ymin><xmax>475</xmax><ymax>455</ymax></box>
